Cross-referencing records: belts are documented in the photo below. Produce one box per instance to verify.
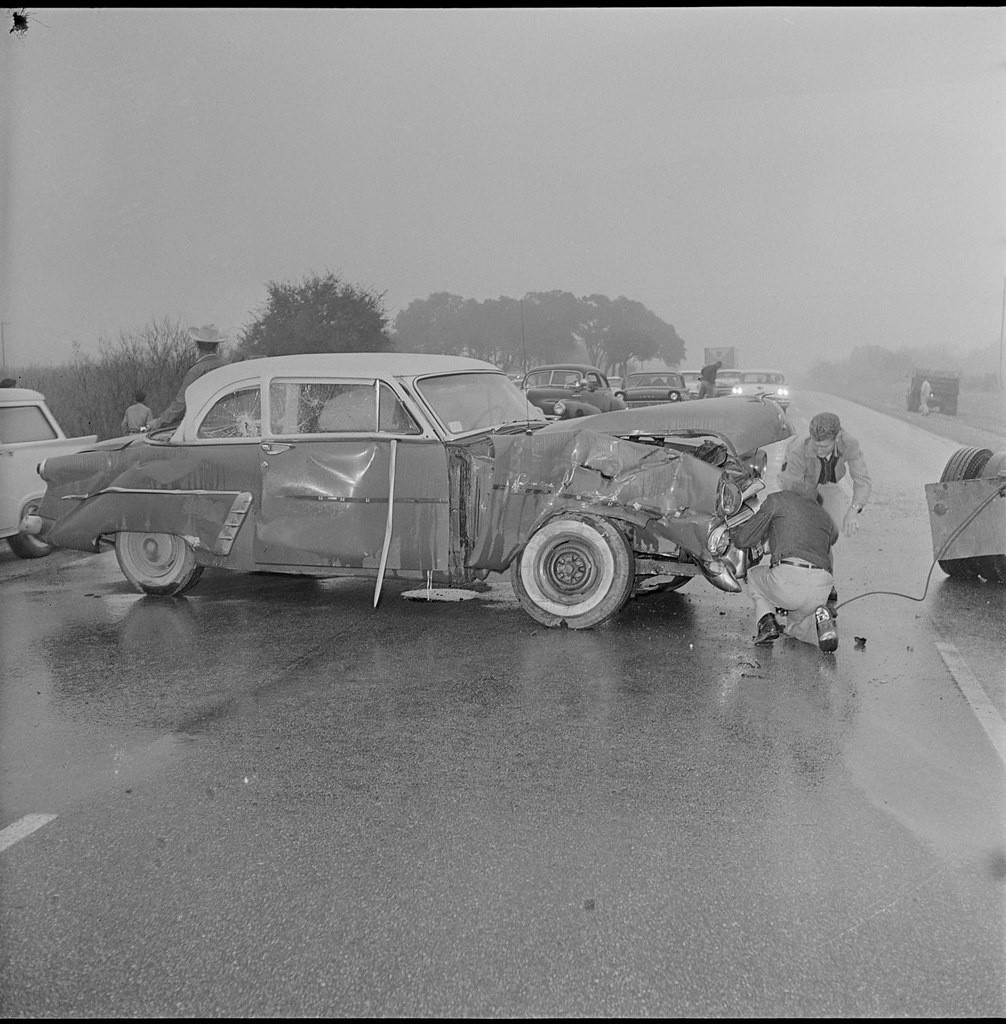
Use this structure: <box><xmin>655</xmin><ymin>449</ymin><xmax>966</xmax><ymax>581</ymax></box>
<box><xmin>774</xmin><ymin>560</ymin><xmax>822</xmax><ymax>570</ymax></box>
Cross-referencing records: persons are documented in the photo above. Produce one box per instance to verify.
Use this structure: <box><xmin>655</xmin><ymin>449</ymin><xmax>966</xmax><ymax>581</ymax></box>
<box><xmin>121</xmin><ymin>392</ymin><xmax>153</xmax><ymax>436</ymax></box>
<box><xmin>921</xmin><ymin>378</ymin><xmax>930</xmax><ymax>416</ymax></box>
<box><xmin>730</xmin><ymin>480</ymin><xmax>839</xmax><ymax>652</ymax></box>
<box><xmin>0</xmin><ymin>379</ymin><xmax>16</xmax><ymax>388</ymax></box>
<box><xmin>776</xmin><ymin>412</ymin><xmax>872</xmax><ymax>616</ymax></box>
<box><xmin>697</xmin><ymin>361</ymin><xmax>723</xmax><ymax>399</ymax></box>
<box><xmin>145</xmin><ymin>323</ymin><xmax>226</xmax><ymax>432</ymax></box>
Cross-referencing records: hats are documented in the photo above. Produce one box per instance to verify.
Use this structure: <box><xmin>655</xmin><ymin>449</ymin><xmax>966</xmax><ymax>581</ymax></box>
<box><xmin>188</xmin><ymin>325</ymin><xmax>225</xmax><ymax>344</ymax></box>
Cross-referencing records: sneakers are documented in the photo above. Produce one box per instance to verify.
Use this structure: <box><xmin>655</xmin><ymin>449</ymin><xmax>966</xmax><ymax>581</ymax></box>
<box><xmin>814</xmin><ymin>605</ymin><xmax>838</xmax><ymax>654</ymax></box>
<box><xmin>753</xmin><ymin>614</ymin><xmax>779</xmax><ymax>645</ymax></box>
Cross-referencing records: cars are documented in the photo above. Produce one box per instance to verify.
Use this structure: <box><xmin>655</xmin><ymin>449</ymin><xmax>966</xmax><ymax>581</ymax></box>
<box><xmin>501</xmin><ymin>369</ymin><xmax>790</xmax><ymax>415</ymax></box>
<box><xmin>19</xmin><ymin>352</ymin><xmax>794</xmax><ymax>631</ymax></box>
<box><xmin>0</xmin><ymin>388</ymin><xmax>98</xmax><ymax>558</ymax></box>
<box><xmin>522</xmin><ymin>364</ymin><xmax>630</xmax><ymax>424</ymax></box>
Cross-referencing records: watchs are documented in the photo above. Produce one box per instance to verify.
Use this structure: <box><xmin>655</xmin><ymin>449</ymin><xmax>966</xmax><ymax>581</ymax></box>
<box><xmin>850</xmin><ymin>505</ymin><xmax>862</xmax><ymax>513</ymax></box>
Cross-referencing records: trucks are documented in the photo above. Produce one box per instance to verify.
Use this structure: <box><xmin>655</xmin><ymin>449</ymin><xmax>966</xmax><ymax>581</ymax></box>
<box><xmin>907</xmin><ymin>369</ymin><xmax>961</xmax><ymax>416</ymax></box>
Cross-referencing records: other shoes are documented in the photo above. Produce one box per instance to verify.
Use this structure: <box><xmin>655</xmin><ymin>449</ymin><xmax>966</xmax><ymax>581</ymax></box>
<box><xmin>921</xmin><ymin>412</ymin><xmax>925</xmax><ymax>416</ymax></box>
<box><xmin>926</xmin><ymin>412</ymin><xmax>929</xmax><ymax>416</ymax></box>
